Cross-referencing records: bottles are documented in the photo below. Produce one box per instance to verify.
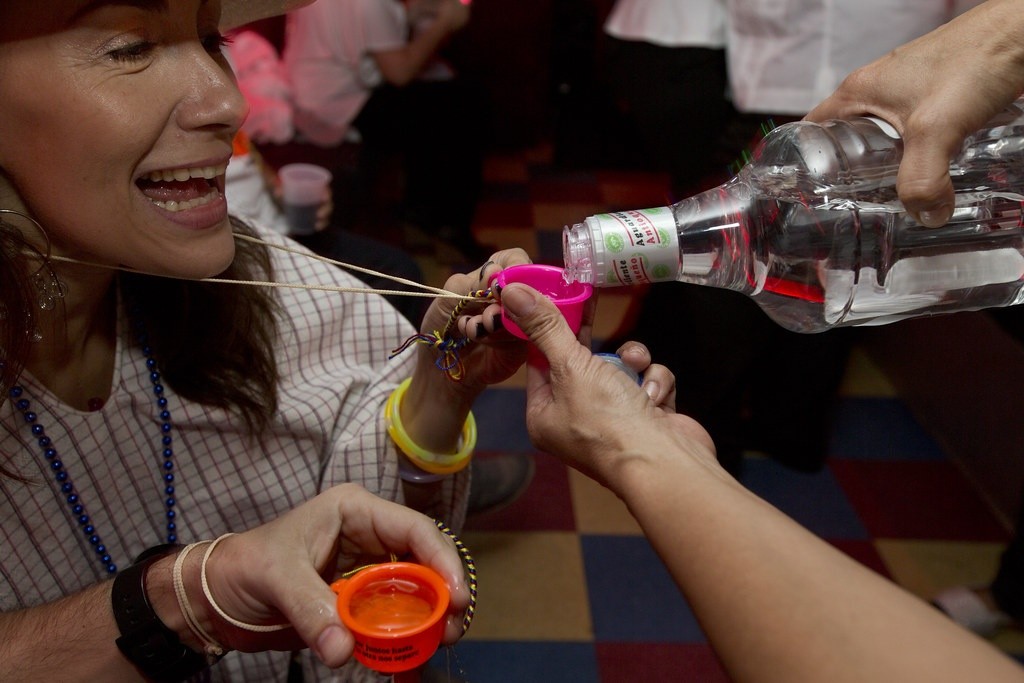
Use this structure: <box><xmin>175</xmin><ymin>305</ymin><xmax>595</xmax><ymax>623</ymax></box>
<box><xmin>564</xmin><ymin>95</ymin><xmax>1024</xmax><ymax>335</ymax></box>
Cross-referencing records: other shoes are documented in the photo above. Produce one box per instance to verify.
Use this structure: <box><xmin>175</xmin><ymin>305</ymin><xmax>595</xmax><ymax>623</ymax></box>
<box><xmin>680</xmin><ymin>391</ymin><xmax>743</xmax><ymax>466</ymax></box>
<box><xmin>420</xmin><ymin>212</ymin><xmax>497</xmax><ymax>266</ymax></box>
<box><xmin>933</xmin><ymin>582</ymin><xmax>1023</xmax><ymax>636</ymax></box>
<box><xmin>746</xmin><ymin>404</ymin><xmax>828</xmax><ymax>473</ymax></box>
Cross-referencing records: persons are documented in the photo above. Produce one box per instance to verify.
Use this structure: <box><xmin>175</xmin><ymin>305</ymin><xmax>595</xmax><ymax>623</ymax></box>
<box><xmin>573</xmin><ymin>0</ymin><xmax>728</xmax><ymax>176</ymax></box>
<box><xmin>1</xmin><ymin>0</ymin><xmax>532</xmax><ymax>683</ymax></box>
<box><xmin>932</xmin><ymin>518</ymin><xmax>1024</xmax><ymax>629</ymax></box>
<box><xmin>500</xmin><ymin>0</ymin><xmax>1024</xmax><ymax>683</ymax></box>
<box><xmin>281</xmin><ymin>0</ymin><xmax>471</xmax><ymax>223</ymax></box>
<box><xmin>669</xmin><ymin>0</ymin><xmax>956</xmax><ymax>196</ymax></box>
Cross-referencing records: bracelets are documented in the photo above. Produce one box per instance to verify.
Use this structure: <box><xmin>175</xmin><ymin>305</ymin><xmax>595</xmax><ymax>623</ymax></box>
<box><xmin>173</xmin><ymin>532</ymin><xmax>293</xmax><ymax>656</ymax></box>
<box><xmin>386</xmin><ymin>377</ymin><xmax>476</xmax><ymax>474</ymax></box>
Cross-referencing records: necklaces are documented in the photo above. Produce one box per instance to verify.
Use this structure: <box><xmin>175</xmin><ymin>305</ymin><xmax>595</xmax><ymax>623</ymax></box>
<box><xmin>14</xmin><ymin>234</ymin><xmax>498</xmax><ymax>350</ymax></box>
<box><xmin>11</xmin><ymin>342</ymin><xmax>177</xmax><ymax>577</ymax></box>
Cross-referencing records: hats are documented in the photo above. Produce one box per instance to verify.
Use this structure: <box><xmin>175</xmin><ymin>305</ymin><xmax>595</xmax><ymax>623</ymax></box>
<box><xmin>218</xmin><ymin>0</ymin><xmax>317</xmax><ymax>38</ymax></box>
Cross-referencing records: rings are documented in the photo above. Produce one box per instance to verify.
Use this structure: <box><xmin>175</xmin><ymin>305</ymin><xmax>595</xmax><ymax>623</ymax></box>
<box><xmin>480</xmin><ymin>261</ymin><xmax>495</xmax><ymax>283</ymax></box>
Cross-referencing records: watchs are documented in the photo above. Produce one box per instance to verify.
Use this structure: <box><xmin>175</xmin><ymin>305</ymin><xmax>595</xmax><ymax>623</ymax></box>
<box><xmin>111</xmin><ymin>543</ymin><xmax>216</xmax><ymax>683</ymax></box>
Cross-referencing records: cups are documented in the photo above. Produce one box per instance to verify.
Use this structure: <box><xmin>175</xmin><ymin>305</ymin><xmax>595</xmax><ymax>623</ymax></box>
<box><xmin>279</xmin><ymin>163</ymin><xmax>332</xmax><ymax>235</ymax></box>
<box><xmin>488</xmin><ymin>264</ymin><xmax>592</xmax><ymax>342</ymax></box>
<box><xmin>329</xmin><ymin>562</ymin><xmax>448</xmax><ymax>673</ymax></box>
